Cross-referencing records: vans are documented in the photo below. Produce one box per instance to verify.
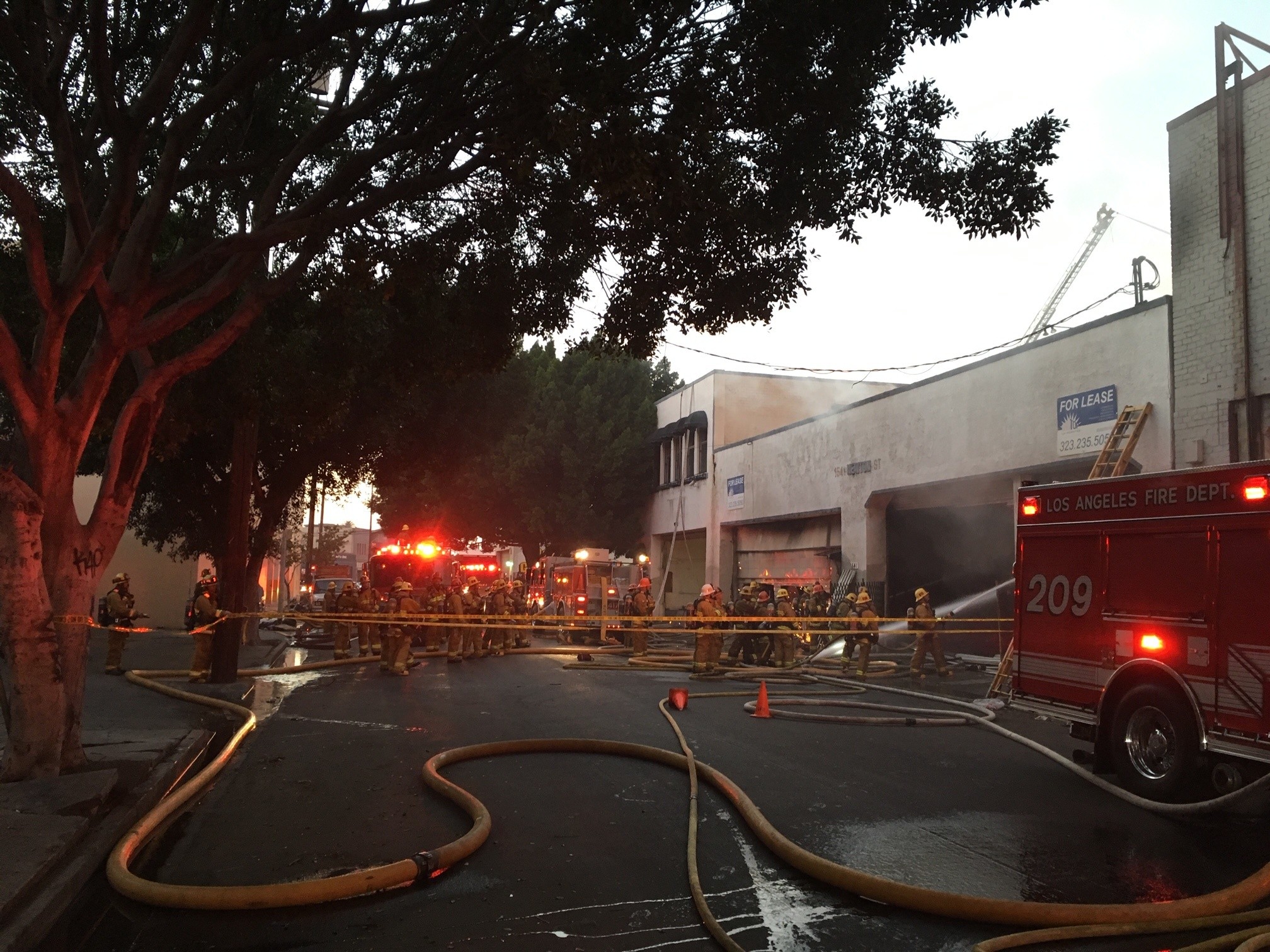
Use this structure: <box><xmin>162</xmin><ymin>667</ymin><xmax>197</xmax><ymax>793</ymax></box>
<box><xmin>307</xmin><ymin>578</ymin><xmax>359</xmax><ymax>614</ymax></box>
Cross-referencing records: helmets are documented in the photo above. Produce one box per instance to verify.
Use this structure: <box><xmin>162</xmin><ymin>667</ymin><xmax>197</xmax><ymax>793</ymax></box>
<box><xmin>638</xmin><ymin>578</ymin><xmax>652</xmax><ymax>587</ymax></box>
<box><xmin>813</xmin><ymin>584</ymin><xmax>825</xmax><ymax>593</ymax></box>
<box><xmin>201</xmin><ymin>568</ymin><xmax>211</xmax><ymax>577</ymax></box>
<box><xmin>858</xmin><ymin>586</ymin><xmax>867</xmax><ymax>593</ymax></box>
<box><xmin>803</xmin><ymin>585</ymin><xmax>814</xmax><ymax>593</ymax></box>
<box><xmin>915</xmin><ymin>588</ymin><xmax>930</xmax><ymax>602</ymax></box>
<box><xmin>699</xmin><ymin>584</ymin><xmax>716</xmax><ymax>596</ymax></box>
<box><xmin>856</xmin><ymin>592</ymin><xmax>872</xmax><ymax>604</ymax></box>
<box><xmin>845</xmin><ymin>592</ymin><xmax>856</xmax><ymax>603</ymax></box>
<box><xmin>711</xmin><ymin>587</ymin><xmax>722</xmax><ymax>598</ymax></box>
<box><xmin>739</xmin><ymin>586</ymin><xmax>752</xmax><ymax>595</ymax></box>
<box><xmin>112</xmin><ymin>572</ymin><xmax>131</xmax><ymax>585</ymax></box>
<box><xmin>750</xmin><ymin>581</ymin><xmax>758</xmax><ymax>588</ymax></box>
<box><xmin>327</xmin><ymin>571</ymin><xmax>524</xmax><ymax>597</ymax></box>
<box><xmin>757</xmin><ymin>590</ymin><xmax>770</xmax><ymax>602</ymax></box>
<box><xmin>776</xmin><ymin>588</ymin><xmax>789</xmax><ymax>597</ymax></box>
<box><xmin>200</xmin><ymin>574</ymin><xmax>219</xmax><ymax>587</ymax></box>
<box><xmin>628</xmin><ymin>583</ymin><xmax>637</xmax><ymax>590</ymax></box>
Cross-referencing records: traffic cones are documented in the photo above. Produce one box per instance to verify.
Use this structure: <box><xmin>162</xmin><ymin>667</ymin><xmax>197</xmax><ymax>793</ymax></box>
<box><xmin>748</xmin><ymin>681</ymin><xmax>773</xmax><ymax>719</ymax></box>
<box><xmin>668</xmin><ymin>688</ymin><xmax>688</xmax><ymax>711</ymax></box>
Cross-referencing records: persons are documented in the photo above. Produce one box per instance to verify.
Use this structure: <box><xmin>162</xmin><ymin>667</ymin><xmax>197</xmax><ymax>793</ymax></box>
<box><xmin>189</xmin><ymin>568</ymin><xmax>232</xmax><ymax>685</ymax></box>
<box><xmin>692</xmin><ymin>582</ymin><xmax>831</xmax><ymax>676</ymax></box>
<box><xmin>911</xmin><ymin>588</ymin><xmax>954</xmax><ymax>677</ymax></box>
<box><xmin>104</xmin><ymin>573</ymin><xmax>143</xmax><ymax>677</ymax></box>
<box><xmin>623</xmin><ymin>577</ymin><xmax>655</xmax><ymax>659</ymax></box>
<box><xmin>320</xmin><ymin>575</ymin><xmax>529</xmax><ymax>676</ymax></box>
<box><xmin>839</xmin><ymin>586</ymin><xmax>881</xmax><ymax>682</ymax></box>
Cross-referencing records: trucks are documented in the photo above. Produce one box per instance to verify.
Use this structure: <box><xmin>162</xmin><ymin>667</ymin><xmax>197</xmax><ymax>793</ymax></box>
<box><xmin>311</xmin><ymin>552</ymin><xmax>359</xmax><ymax>583</ymax></box>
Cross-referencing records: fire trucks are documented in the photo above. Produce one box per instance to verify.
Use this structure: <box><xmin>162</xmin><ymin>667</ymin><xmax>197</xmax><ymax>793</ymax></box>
<box><xmin>517</xmin><ymin>547</ymin><xmax>652</xmax><ymax>645</ymax></box>
<box><xmin>1008</xmin><ymin>459</ymin><xmax>1269</xmax><ymax>805</ymax></box>
<box><xmin>363</xmin><ymin>529</ymin><xmax>503</xmax><ymax>616</ymax></box>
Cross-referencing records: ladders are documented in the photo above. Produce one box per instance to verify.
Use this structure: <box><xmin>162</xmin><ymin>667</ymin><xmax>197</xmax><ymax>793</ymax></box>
<box><xmin>984</xmin><ymin>400</ymin><xmax>1154</xmax><ymax>704</ymax></box>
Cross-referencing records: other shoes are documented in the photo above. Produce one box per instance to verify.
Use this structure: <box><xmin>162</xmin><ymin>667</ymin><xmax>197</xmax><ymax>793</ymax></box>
<box><xmin>691</xmin><ymin>656</ymin><xmax>865</xmax><ymax>682</ymax></box>
<box><xmin>105</xmin><ymin>668</ymin><xmax>126</xmax><ymax>676</ymax></box>
<box><xmin>939</xmin><ymin>668</ymin><xmax>955</xmax><ymax>676</ymax></box>
<box><xmin>189</xmin><ymin>677</ymin><xmax>210</xmax><ymax>683</ymax></box>
<box><xmin>910</xmin><ymin>672</ymin><xmax>922</xmax><ymax>677</ymax></box>
<box><xmin>332</xmin><ymin>640</ymin><xmax>530</xmax><ymax>674</ymax></box>
